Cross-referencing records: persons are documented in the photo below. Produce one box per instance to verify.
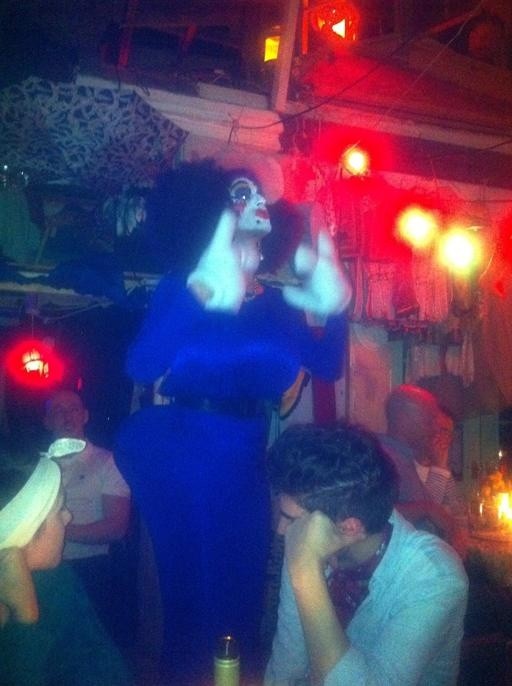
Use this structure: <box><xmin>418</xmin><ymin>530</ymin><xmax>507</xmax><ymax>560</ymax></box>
<box><xmin>415</xmin><ymin>407</ymin><xmax>463</xmax><ymax>507</ymax></box>
<box><xmin>113</xmin><ymin>167</ymin><xmax>358</xmax><ymax>686</ymax></box>
<box><xmin>36</xmin><ymin>389</ymin><xmax>133</xmax><ymax>654</ymax></box>
<box><xmin>261</xmin><ymin>413</ymin><xmax>471</xmax><ymax>686</ymax></box>
<box><xmin>377</xmin><ymin>382</ymin><xmax>457</xmax><ymax>539</ymax></box>
<box><xmin>1</xmin><ymin>437</ymin><xmax>133</xmax><ymax>685</ymax></box>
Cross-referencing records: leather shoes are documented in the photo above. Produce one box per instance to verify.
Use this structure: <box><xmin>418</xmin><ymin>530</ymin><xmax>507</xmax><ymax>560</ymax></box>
<box><xmin>279</xmin><ymin>367</ymin><xmax>311</xmax><ymax>420</ymax></box>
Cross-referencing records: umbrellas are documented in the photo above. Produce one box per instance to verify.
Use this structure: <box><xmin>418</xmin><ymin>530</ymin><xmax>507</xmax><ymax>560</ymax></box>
<box><xmin>0</xmin><ymin>77</ymin><xmax>191</xmax><ymax>199</ymax></box>
